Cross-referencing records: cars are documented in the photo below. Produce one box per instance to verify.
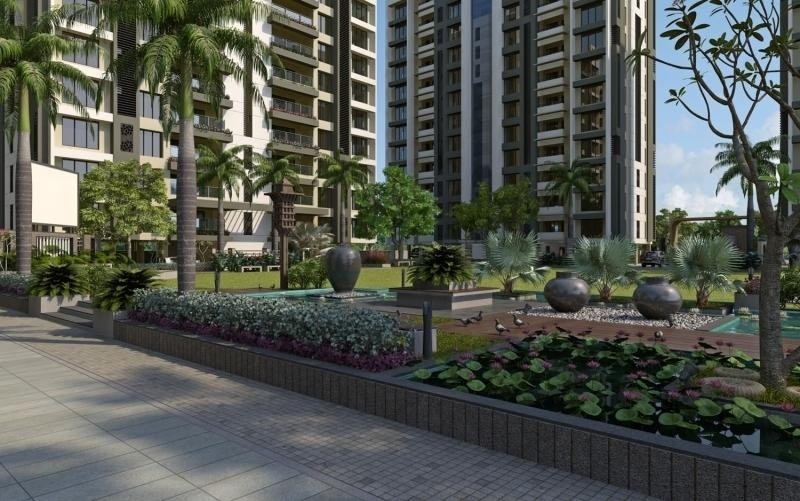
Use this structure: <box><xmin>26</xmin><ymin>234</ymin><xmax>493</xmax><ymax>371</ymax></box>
<box><xmin>640</xmin><ymin>250</ymin><xmax>664</xmax><ymax>267</ymax></box>
